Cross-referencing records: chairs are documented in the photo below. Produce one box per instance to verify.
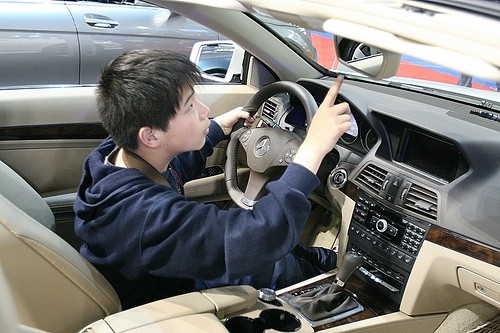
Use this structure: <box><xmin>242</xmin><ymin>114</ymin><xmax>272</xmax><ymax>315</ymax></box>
<box><xmin>0</xmin><ymin>159</ymin><xmax>123</xmax><ymax>332</ymax></box>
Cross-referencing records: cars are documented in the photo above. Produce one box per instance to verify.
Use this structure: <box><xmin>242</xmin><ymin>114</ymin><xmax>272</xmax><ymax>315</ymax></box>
<box><xmin>0</xmin><ymin>1</ymin><xmax>319</xmax><ymax>88</ymax></box>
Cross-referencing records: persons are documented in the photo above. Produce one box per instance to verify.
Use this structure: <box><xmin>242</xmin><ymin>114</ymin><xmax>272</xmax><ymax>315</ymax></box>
<box><xmin>72</xmin><ymin>48</ymin><xmax>352</xmax><ymax>312</ymax></box>
